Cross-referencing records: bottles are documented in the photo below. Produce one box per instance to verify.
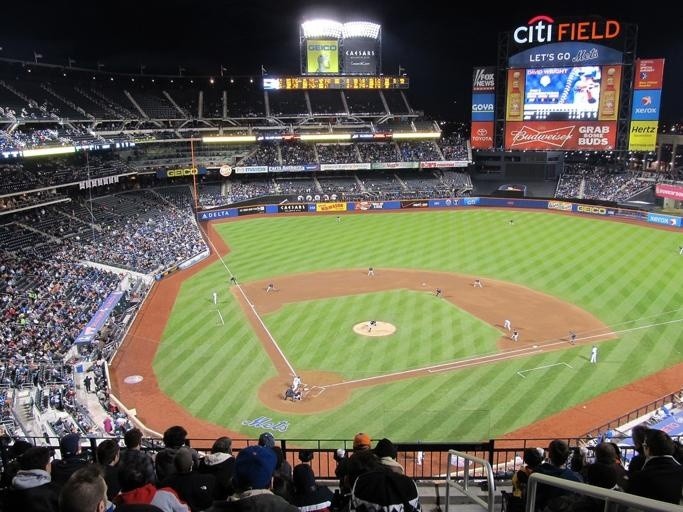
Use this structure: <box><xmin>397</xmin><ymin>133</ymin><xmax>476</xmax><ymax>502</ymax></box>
<box><xmin>600</xmin><ymin>73</ymin><xmax>616</xmax><ymax>117</ymax></box>
<box><xmin>507</xmin><ymin>80</ymin><xmax>521</xmax><ymax>117</ymax></box>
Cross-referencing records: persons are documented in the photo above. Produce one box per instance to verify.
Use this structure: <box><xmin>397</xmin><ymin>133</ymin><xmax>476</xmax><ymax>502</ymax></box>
<box><xmin>567</xmin><ymin>327</ymin><xmax>576</xmax><ymax>344</ymax></box>
<box><xmin>556</xmin><ymin>153</ymin><xmax>664</xmax><ymax>207</ymax></box>
<box><xmin>129</xmin><ymin>129</ymin><xmax>205</xmax><ymax>170</ymax></box>
<box><xmin>265</xmin><ymin>281</ymin><xmax>273</xmax><ymax>293</ymax></box>
<box><xmin>336</xmin><ymin>214</ymin><xmax>342</xmax><ymax>222</ymax></box>
<box><xmin>114</xmin><ymin>424</ymin><xmax>336</xmax><ymax>511</ymax></box>
<box><xmin>510</xmin><ymin>328</ymin><xmax>518</xmax><ymax>341</ymax></box>
<box><xmin>503</xmin><ymin>320</ymin><xmax>511</xmax><ymax>330</ymax></box>
<box><xmin>230</xmin><ymin>275</ymin><xmax>237</xmax><ymax>283</ymax></box>
<box><xmin>591</xmin><ymin>344</ymin><xmax>598</xmax><ymax>363</ymax></box>
<box><xmin>283</xmin><ymin>375</ymin><xmax>304</xmax><ymax>402</ymax></box>
<box><xmin>1</xmin><ymin>60</ymin><xmax>206</xmax><ymax>421</ymax></box>
<box><xmin>333</xmin><ymin>432</ymin><xmax>421</xmax><ymax>511</ymax></box>
<box><xmin>2</xmin><ymin>100</ymin><xmax>48</xmax><ymax>121</ymax></box>
<box><xmin>368</xmin><ymin>320</ymin><xmax>376</xmax><ymax>333</ymax></box>
<box><xmin>207</xmin><ymin>82</ymin><xmax>472</xmax><ymax>205</ymax></box>
<box><xmin>506</xmin><ymin>218</ymin><xmax>514</xmax><ymax>225</ymax></box>
<box><xmin>1</xmin><ymin>427</ymin><xmax>154</xmax><ymax>511</ymax></box>
<box><xmin>502</xmin><ymin>425</ymin><xmax>683</xmax><ymax>510</ymax></box>
<box><xmin>473</xmin><ymin>278</ymin><xmax>483</xmax><ymax>287</ymax></box>
<box><xmin>433</xmin><ymin>287</ymin><xmax>444</xmax><ymax>297</ymax></box>
<box><xmin>212</xmin><ymin>291</ymin><xmax>218</xmax><ymax>305</ymax></box>
<box><xmin>677</xmin><ymin>246</ymin><xmax>683</xmax><ymax>255</ymax></box>
<box><xmin>367</xmin><ymin>265</ymin><xmax>376</xmax><ymax>278</ymax></box>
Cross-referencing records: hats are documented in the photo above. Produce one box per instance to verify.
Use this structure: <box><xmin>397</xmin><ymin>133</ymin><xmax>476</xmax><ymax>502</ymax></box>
<box><xmin>232</xmin><ymin>446</ymin><xmax>278</xmax><ymax>489</ymax></box>
<box><xmin>354</xmin><ymin>432</ymin><xmax>371</xmax><ymax>448</ymax></box>
<box><xmin>374</xmin><ymin>439</ymin><xmax>396</xmax><ymax>457</ymax></box>
<box><xmin>174</xmin><ymin>448</ymin><xmax>193</xmax><ymax>470</ymax></box>
<box><xmin>59</xmin><ymin>434</ymin><xmax>80</xmax><ymax>457</ymax></box>
<box><xmin>211</xmin><ymin>437</ymin><xmax>231</xmax><ymax>454</ymax></box>
<box><xmin>258</xmin><ymin>432</ymin><xmax>275</xmax><ymax>446</ymax></box>
<box><xmin>97</xmin><ymin>439</ymin><xmax>119</xmax><ymax>465</ymax></box>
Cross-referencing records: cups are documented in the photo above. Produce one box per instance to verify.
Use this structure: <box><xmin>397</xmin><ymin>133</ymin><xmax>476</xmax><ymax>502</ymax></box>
<box><xmin>578</xmin><ymin>447</ymin><xmax>589</xmax><ymax>457</ymax></box>
<box><xmin>537</xmin><ymin>447</ymin><xmax>545</xmax><ymax>458</ymax></box>
<box><xmin>336</xmin><ymin>448</ymin><xmax>345</xmax><ymax>459</ymax></box>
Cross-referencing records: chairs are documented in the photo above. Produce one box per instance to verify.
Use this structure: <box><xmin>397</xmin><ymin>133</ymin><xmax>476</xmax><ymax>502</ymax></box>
<box><xmin>0</xmin><ymin>121</ymin><xmax>200</xmax><ymax>439</ymax></box>
<box><xmin>200</xmin><ymin>120</ymin><xmax>475</xmax><ymax>210</ymax></box>
<box><xmin>555</xmin><ymin>161</ymin><xmax>667</xmax><ymax>207</ymax></box>
<box><xmin>0</xmin><ymin>59</ymin><xmax>415</xmax><ymax>118</ymax></box>
